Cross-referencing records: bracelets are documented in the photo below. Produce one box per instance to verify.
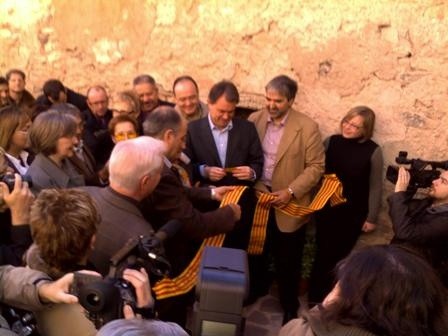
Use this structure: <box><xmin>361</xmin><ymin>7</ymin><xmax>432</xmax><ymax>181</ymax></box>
<box><xmin>288</xmin><ymin>187</ymin><xmax>295</xmax><ymax>199</ymax></box>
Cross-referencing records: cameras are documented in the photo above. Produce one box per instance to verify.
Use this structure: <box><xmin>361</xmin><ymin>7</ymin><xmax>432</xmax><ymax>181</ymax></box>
<box><xmin>0</xmin><ymin>174</ymin><xmax>33</xmax><ymax>193</ymax></box>
<box><xmin>70</xmin><ymin>272</ymin><xmax>136</xmax><ymax>315</ymax></box>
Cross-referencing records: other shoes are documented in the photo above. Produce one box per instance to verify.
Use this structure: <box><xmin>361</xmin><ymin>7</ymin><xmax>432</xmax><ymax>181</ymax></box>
<box><xmin>243</xmin><ymin>288</ymin><xmax>269</xmax><ymax>308</ymax></box>
<box><xmin>282</xmin><ymin>310</ymin><xmax>298</xmax><ymax>327</ymax></box>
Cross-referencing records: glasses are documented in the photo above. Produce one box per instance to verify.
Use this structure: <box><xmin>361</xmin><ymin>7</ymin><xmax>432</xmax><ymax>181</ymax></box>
<box><xmin>342</xmin><ymin>120</ymin><xmax>362</xmax><ymax>133</ymax></box>
<box><xmin>87</xmin><ymin>98</ymin><xmax>108</xmax><ymax>106</ymax></box>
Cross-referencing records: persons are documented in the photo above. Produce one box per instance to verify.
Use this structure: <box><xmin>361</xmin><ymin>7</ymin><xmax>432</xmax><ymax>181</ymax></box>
<box><xmin>0</xmin><ymin>69</ymin><xmax>448</xmax><ymax>336</ymax></box>
